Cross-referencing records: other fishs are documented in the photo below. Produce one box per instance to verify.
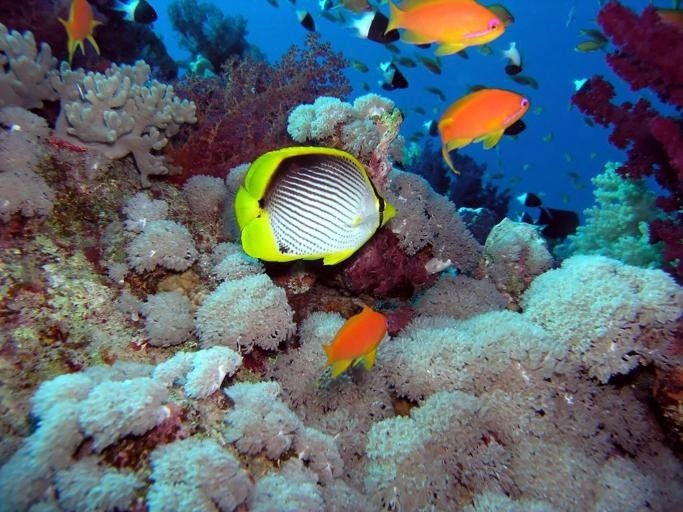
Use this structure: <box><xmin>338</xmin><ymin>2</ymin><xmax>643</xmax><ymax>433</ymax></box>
<box><xmin>176</xmin><ymin>2</ymin><xmax>617</xmax><ymax>269</ymax></box>
